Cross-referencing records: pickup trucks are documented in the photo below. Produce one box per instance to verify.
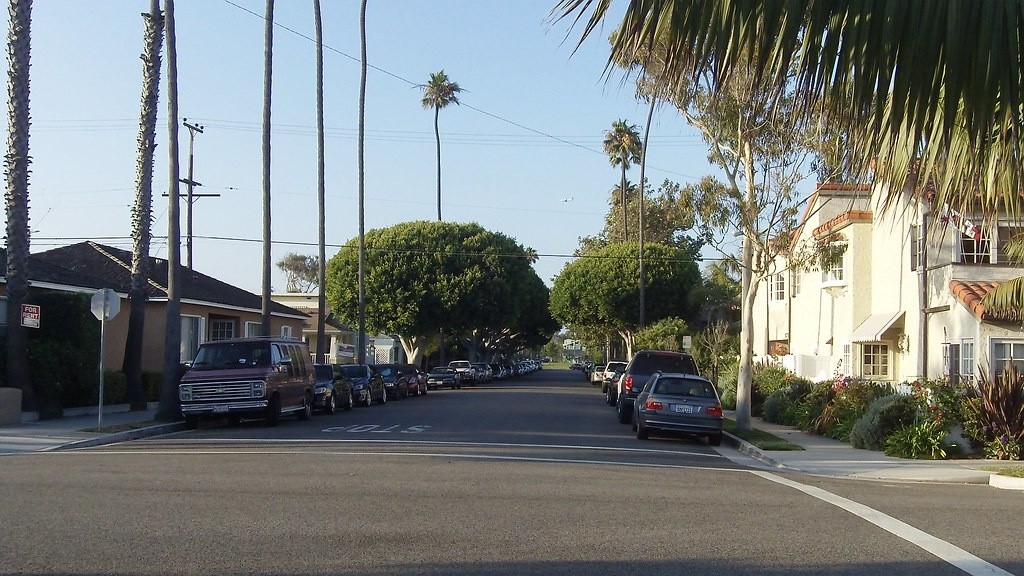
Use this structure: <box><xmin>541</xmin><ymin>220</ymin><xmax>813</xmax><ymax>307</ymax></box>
<box><xmin>447</xmin><ymin>361</ymin><xmax>477</xmax><ymax>388</ymax></box>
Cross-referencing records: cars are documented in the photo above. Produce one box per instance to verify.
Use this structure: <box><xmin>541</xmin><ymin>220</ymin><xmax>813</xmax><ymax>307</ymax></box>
<box><xmin>340</xmin><ymin>363</ymin><xmax>387</xmax><ymax>408</ymax></box>
<box><xmin>427</xmin><ymin>367</ymin><xmax>461</xmax><ymax>390</ymax></box>
<box><xmin>630</xmin><ymin>370</ymin><xmax>724</xmax><ymax>447</ymax></box>
<box><xmin>471</xmin><ymin>361</ymin><xmax>494</xmax><ymax>385</ymax></box>
<box><xmin>401</xmin><ymin>364</ymin><xmax>428</xmax><ymax>397</ymax></box>
<box><xmin>373</xmin><ymin>363</ymin><xmax>410</xmax><ymax>401</ymax></box>
<box><xmin>488</xmin><ymin>353</ymin><xmax>550</xmax><ymax>382</ymax></box>
<box><xmin>308</xmin><ymin>363</ymin><xmax>356</xmax><ymax>417</ymax></box>
<box><xmin>565</xmin><ymin>356</ymin><xmax>630</xmax><ymax>406</ymax></box>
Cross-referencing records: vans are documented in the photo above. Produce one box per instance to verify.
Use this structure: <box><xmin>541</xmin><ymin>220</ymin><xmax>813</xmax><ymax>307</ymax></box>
<box><xmin>177</xmin><ymin>334</ymin><xmax>315</xmax><ymax>427</ymax></box>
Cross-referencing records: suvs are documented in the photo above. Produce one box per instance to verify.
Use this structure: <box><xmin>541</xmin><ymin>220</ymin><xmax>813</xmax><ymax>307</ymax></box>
<box><xmin>613</xmin><ymin>349</ymin><xmax>703</xmax><ymax>425</ymax></box>
